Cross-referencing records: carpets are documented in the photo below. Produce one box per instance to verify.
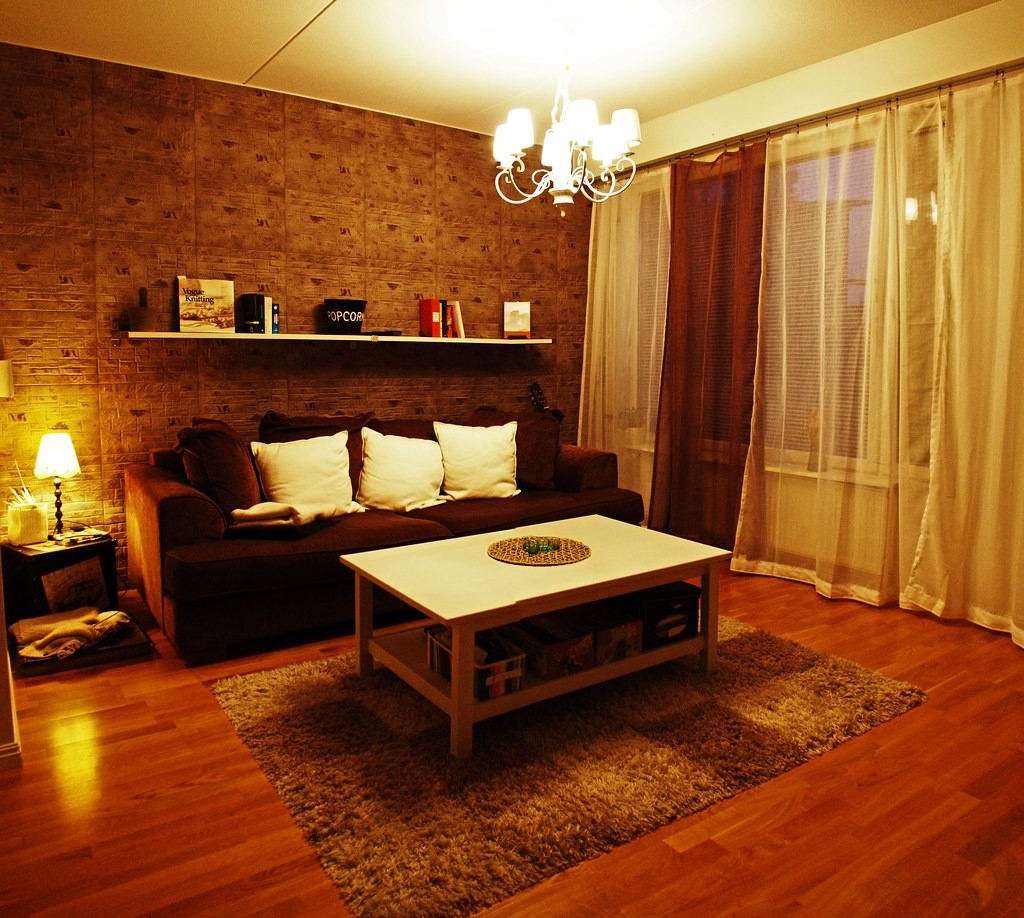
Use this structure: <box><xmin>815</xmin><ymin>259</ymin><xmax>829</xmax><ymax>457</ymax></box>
<box><xmin>202</xmin><ymin>607</ymin><xmax>932</xmax><ymax>916</ymax></box>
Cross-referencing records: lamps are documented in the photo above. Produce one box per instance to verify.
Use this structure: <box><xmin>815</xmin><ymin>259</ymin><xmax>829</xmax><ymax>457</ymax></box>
<box><xmin>492</xmin><ymin>86</ymin><xmax>641</xmax><ymax>219</ymax></box>
<box><xmin>33</xmin><ymin>433</ymin><xmax>83</xmax><ymax>538</ymax></box>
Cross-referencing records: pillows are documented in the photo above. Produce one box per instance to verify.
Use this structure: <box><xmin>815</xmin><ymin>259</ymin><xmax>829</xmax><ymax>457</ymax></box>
<box><xmin>355</xmin><ymin>426</ymin><xmax>446</xmax><ymax>514</ymax></box>
<box><xmin>433</xmin><ymin>420</ymin><xmax>522</xmax><ymax>502</ymax></box>
<box><xmin>248</xmin><ymin>430</ymin><xmax>364</xmax><ymax>527</ymax></box>
<box><xmin>174</xmin><ymin>416</ymin><xmax>261</xmax><ymax>524</ymax></box>
<box><xmin>367</xmin><ymin>420</ymin><xmax>444</xmax><ymax>442</ymax></box>
<box><xmin>258</xmin><ymin>411</ymin><xmax>375</xmax><ymax>503</ymax></box>
<box><xmin>470</xmin><ymin>407</ymin><xmax>564</xmax><ymax>495</ymax></box>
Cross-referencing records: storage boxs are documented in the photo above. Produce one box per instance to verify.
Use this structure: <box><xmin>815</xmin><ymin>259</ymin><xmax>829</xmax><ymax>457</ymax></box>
<box><xmin>495</xmin><ymin>611</ymin><xmax>596</xmax><ymax>683</ymax></box>
<box><xmin>623</xmin><ymin>580</ymin><xmax>702</xmax><ymax>652</ymax></box>
<box><xmin>574</xmin><ymin>593</ymin><xmax>645</xmax><ymax>667</ymax></box>
<box><xmin>425</xmin><ymin>628</ymin><xmax>526</xmax><ymax>701</ymax></box>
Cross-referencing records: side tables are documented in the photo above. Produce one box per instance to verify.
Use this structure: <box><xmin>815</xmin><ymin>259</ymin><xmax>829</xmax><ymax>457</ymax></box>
<box><xmin>2</xmin><ymin>529</ymin><xmax>120</xmax><ymax>618</ymax></box>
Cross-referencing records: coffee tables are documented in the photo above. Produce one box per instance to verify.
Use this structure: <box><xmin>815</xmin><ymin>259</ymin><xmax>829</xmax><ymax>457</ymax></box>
<box><xmin>340</xmin><ymin>513</ymin><xmax>733</xmax><ymax>758</ymax></box>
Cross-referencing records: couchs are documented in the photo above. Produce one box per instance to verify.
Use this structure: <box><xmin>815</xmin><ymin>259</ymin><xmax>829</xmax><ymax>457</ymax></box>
<box><xmin>124</xmin><ymin>442</ymin><xmax>647</xmax><ymax>669</ymax></box>
